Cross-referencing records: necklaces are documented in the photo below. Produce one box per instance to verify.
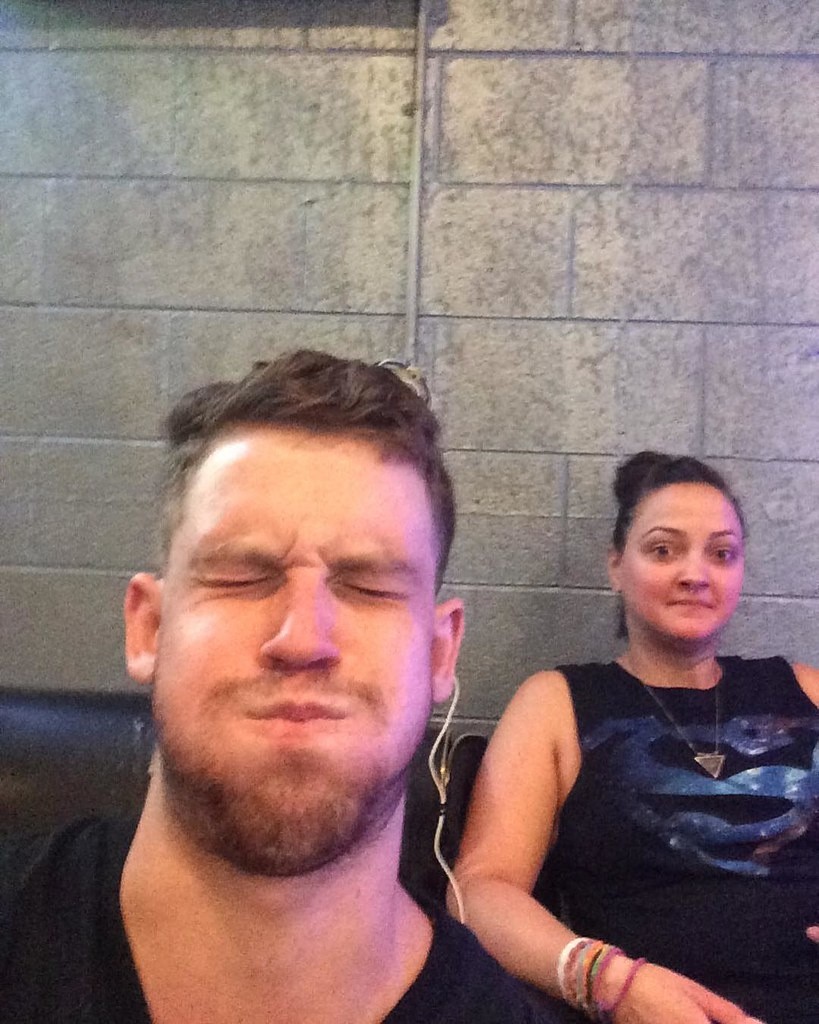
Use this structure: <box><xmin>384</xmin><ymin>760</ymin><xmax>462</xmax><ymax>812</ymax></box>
<box><xmin>622</xmin><ymin>652</ymin><xmax>726</xmax><ymax>781</ymax></box>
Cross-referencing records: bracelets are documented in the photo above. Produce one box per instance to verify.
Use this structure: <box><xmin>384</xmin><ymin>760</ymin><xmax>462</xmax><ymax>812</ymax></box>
<box><xmin>557</xmin><ymin>936</ymin><xmax>649</xmax><ymax>1024</ymax></box>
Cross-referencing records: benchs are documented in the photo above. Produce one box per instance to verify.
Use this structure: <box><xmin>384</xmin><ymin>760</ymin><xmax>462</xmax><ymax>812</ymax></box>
<box><xmin>1</xmin><ymin>684</ymin><xmax>564</xmax><ymax>920</ymax></box>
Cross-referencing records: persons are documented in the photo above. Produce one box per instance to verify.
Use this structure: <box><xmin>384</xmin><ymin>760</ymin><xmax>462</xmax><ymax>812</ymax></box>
<box><xmin>447</xmin><ymin>449</ymin><xmax>819</xmax><ymax>1024</ymax></box>
<box><xmin>0</xmin><ymin>350</ymin><xmax>585</xmax><ymax>1024</ymax></box>
